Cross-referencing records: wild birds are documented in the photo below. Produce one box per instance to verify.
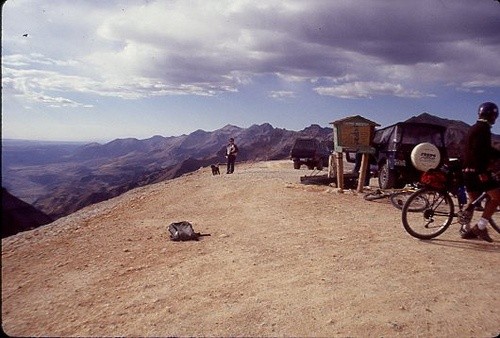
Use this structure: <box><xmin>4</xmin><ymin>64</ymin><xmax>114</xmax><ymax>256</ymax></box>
<box><xmin>21</xmin><ymin>33</ymin><xmax>28</xmax><ymax>37</ymax></box>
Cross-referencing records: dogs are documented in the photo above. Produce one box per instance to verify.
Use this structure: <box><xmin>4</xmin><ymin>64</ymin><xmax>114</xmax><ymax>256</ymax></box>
<box><xmin>210</xmin><ymin>162</ymin><xmax>221</xmax><ymax>176</ymax></box>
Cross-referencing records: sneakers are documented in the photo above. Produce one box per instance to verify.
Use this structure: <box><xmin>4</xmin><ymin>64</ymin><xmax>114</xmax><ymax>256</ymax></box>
<box><xmin>461</xmin><ymin>225</ymin><xmax>474</xmax><ymax>236</ymax></box>
<box><xmin>472</xmin><ymin>224</ymin><xmax>493</xmax><ymax>243</ymax></box>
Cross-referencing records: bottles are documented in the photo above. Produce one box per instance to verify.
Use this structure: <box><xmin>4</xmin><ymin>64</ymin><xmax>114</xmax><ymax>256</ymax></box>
<box><xmin>458</xmin><ymin>187</ymin><xmax>468</xmax><ymax>204</ymax></box>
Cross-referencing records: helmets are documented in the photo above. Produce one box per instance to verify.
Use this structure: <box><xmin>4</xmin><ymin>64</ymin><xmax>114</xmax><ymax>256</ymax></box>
<box><xmin>478</xmin><ymin>102</ymin><xmax>498</xmax><ymax>124</ymax></box>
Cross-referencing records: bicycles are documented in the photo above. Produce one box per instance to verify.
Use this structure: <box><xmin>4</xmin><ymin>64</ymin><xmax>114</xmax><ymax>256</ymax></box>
<box><xmin>401</xmin><ymin>152</ymin><xmax>500</xmax><ymax>239</ymax></box>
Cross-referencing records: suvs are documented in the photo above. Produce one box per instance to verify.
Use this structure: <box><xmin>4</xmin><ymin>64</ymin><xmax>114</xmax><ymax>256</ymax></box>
<box><xmin>353</xmin><ymin>119</ymin><xmax>450</xmax><ymax>190</ymax></box>
<box><xmin>289</xmin><ymin>136</ymin><xmax>334</xmax><ymax>170</ymax></box>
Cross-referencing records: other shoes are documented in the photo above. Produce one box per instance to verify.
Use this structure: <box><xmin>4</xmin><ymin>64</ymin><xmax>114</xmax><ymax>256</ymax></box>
<box><xmin>473</xmin><ymin>206</ymin><xmax>484</xmax><ymax>211</ymax></box>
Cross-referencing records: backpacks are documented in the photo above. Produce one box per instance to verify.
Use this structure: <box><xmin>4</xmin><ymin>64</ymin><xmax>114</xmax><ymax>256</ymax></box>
<box><xmin>167</xmin><ymin>221</ymin><xmax>199</xmax><ymax>241</ymax></box>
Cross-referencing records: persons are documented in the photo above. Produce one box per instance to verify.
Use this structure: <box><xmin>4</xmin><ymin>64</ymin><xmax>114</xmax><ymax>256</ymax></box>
<box><xmin>225</xmin><ymin>138</ymin><xmax>239</xmax><ymax>175</ymax></box>
<box><xmin>458</xmin><ymin>102</ymin><xmax>500</xmax><ymax>243</ymax></box>
